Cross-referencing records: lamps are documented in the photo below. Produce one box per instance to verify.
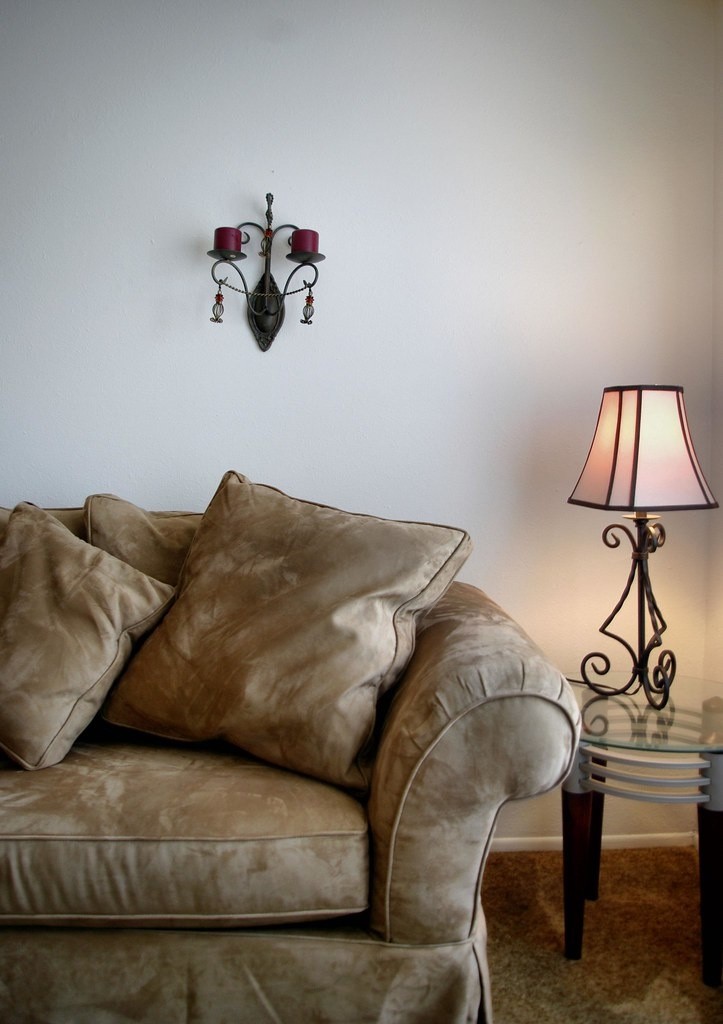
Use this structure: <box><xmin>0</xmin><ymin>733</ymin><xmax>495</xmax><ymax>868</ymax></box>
<box><xmin>566</xmin><ymin>384</ymin><xmax>720</xmax><ymax>710</ymax></box>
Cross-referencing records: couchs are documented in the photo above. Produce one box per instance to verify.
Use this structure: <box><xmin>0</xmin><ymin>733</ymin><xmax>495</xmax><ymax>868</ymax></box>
<box><xmin>0</xmin><ymin>510</ymin><xmax>582</xmax><ymax>1024</ymax></box>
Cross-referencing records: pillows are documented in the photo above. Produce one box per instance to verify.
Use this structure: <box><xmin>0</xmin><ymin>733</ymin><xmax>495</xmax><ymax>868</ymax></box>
<box><xmin>102</xmin><ymin>471</ymin><xmax>475</xmax><ymax>790</ymax></box>
<box><xmin>0</xmin><ymin>502</ymin><xmax>180</xmax><ymax>772</ymax></box>
<box><xmin>82</xmin><ymin>491</ymin><xmax>206</xmax><ymax>584</ymax></box>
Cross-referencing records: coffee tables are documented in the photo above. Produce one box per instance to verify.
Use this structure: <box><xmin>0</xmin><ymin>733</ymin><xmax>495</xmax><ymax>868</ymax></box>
<box><xmin>558</xmin><ymin>672</ymin><xmax>723</xmax><ymax>991</ymax></box>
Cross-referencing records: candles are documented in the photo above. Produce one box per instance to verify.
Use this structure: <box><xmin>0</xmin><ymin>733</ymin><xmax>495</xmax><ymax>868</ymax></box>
<box><xmin>214</xmin><ymin>227</ymin><xmax>242</xmax><ymax>253</ymax></box>
<box><xmin>291</xmin><ymin>229</ymin><xmax>319</xmax><ymax>254</ymax></box>
